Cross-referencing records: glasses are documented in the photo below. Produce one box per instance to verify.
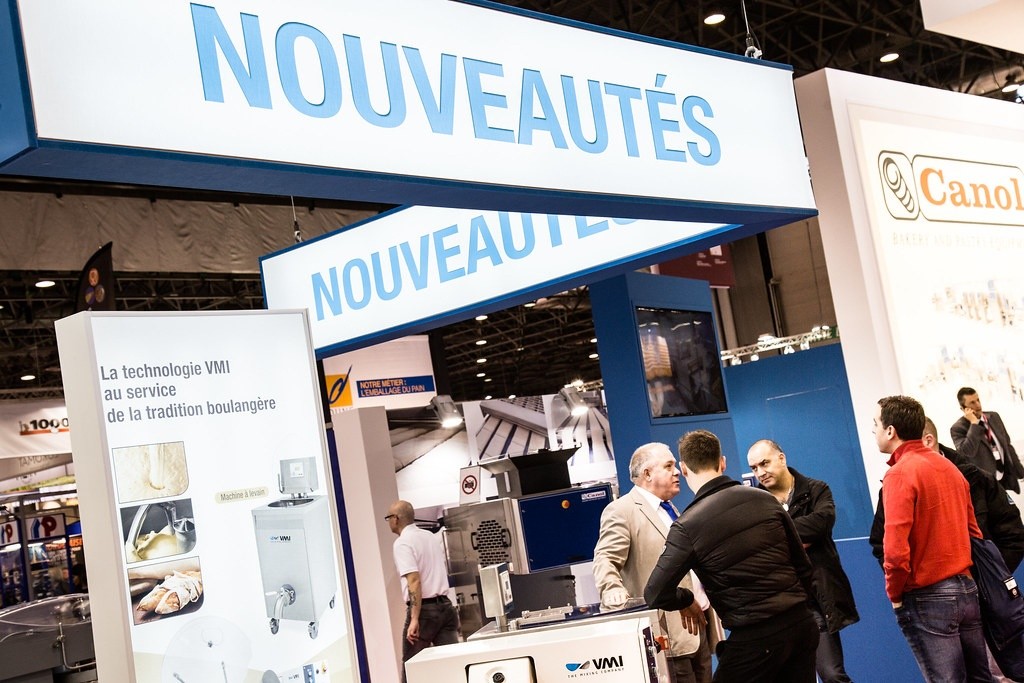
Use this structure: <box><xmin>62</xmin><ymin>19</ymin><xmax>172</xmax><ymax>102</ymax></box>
<box><xmin>384</xmin><ymin>514</ymin><xmax>399</xmax><ymax>521</ymax></box>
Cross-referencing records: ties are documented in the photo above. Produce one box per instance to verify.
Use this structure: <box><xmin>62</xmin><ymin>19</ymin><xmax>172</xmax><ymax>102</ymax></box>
<box><xmin>978</xmin><ymin>420</ymin><xmax>1004</xmax><ymax>472</ymax></box>
<box><xmin>660</xmin><ymin>502</ymin><xmax>678</xmax><ymax>521</ymax></box>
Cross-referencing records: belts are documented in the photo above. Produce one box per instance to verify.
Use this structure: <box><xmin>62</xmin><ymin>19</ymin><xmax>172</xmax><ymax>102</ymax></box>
<box><xmin>406</xmin><ymin>595</ymin><xmax>449</xmax><ymax>606</ymax></box>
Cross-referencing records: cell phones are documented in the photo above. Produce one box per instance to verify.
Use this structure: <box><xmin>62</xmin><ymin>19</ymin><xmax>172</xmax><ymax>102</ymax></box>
<box><xmin>961</xmin><ymin>405</ymin><xmax>966</xmax><ymax>409</ymax></box>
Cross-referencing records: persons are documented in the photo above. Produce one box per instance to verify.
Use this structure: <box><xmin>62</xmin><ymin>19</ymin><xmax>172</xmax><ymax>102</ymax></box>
<box><xmin>384</xmin><ymin>501</ymin><xmax>459</xmax><ymax>683</ymax></box>
<box><xmin>872</xmin><ymin>396</ymin><xmax>993</xmax><ymax>683</ymax></box>
<box><xmin>746</xmin><ymin>438</ymin><xmax>860</xmax><ymax>683</ymax></box>
<box><xmin>868</xmin><ymin>417</ymin><xmax>1024</xmax><ymax>575</ymax></box>
<box><xmin>644</xmin><ymin>431</ymin><xmax>821</xmax><ymax>683</ymax></box>
<box><xmin>592</xmin><ymin>442</ymin><xmax>726</xmax><ymax>683</ymax></box>
<box><xmin>950</xmin><ymin>388</ymin><xmax>1024</xmax><ymax>494</ymax></box>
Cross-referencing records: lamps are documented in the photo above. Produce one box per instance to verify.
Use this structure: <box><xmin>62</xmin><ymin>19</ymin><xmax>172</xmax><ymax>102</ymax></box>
<box><xmin>1002</xmin><ymin>63</ymin><xmax>1019</xmax><ymax>93</ymax></box>
<box><xmin>719</xmin><ymin>323</ymin><xmax>832</xmax><ymax>367</ymax></box>
<box><xmin>426</xmin><ymin>395</ymin><xmax>462</xmax><ymax>428</ymax></box>
<box><xmin>879</xmin><ymin>33</ymin><xmax>899</xmax><ymax>62</ymax></box>
<box><xmin>558</xmin><ymin>386</ymin><xmax>599</xmax><ymax>417</ymax></box>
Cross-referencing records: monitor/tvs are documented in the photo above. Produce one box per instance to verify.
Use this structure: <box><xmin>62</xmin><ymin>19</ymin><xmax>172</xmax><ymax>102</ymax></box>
<box><xmin>634</xmin><ymin>307</ymin><xmax>728</xmax><ymax>419</ymax></box>
